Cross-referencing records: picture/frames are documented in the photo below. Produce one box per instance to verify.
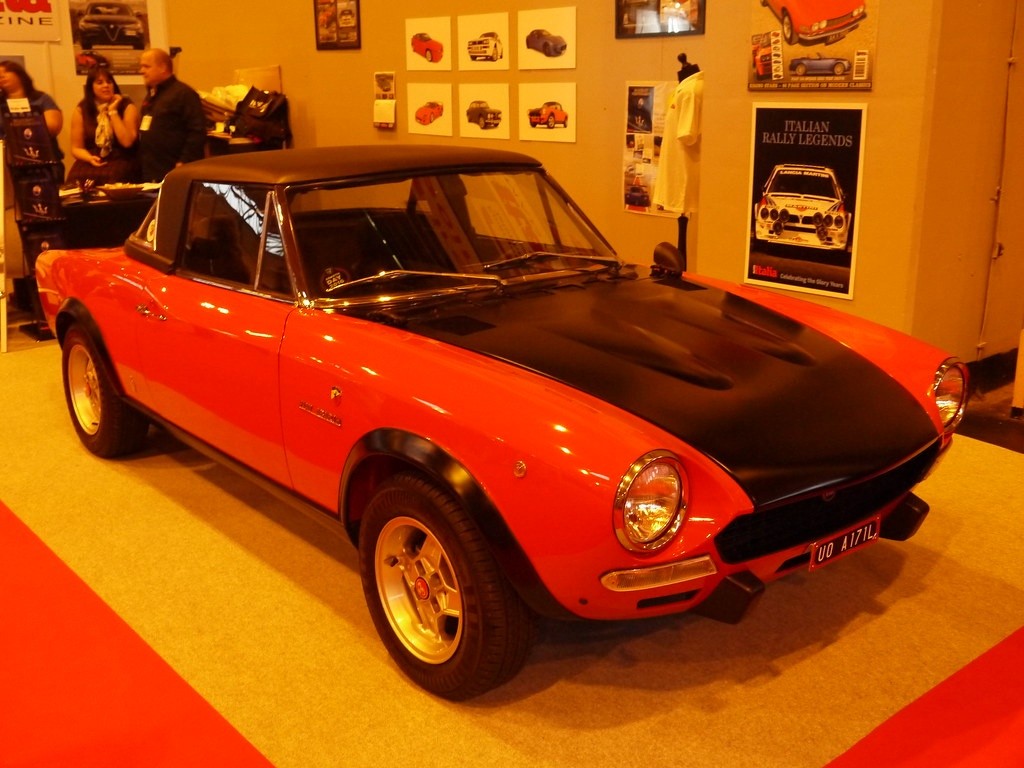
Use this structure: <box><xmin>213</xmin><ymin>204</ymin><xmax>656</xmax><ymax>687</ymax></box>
<box><xmin>314</xmin><ymin>0</ymin><xmax>361</xmax><ymax>51</ymax></box>
<box><xmin>615</xmin><ymin>0</ymin><xmax>706</xmax><ymax>39</ymax></box>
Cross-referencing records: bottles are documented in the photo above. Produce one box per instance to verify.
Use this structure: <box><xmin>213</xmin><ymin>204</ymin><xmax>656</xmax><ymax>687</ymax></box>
<box><xmin>223</xmin><ymin>112</ymin><xmax>231</xmax><ymax>134</ymax></box>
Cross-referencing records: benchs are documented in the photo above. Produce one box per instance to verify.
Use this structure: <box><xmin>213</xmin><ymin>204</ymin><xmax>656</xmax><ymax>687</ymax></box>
<box><xmin>195</xmin><ymin>205</ymin><xmax>486</xmax><ymax>302</ymax></box>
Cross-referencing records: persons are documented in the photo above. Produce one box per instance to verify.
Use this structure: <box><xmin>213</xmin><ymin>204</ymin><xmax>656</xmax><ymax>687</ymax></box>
<box><xmin>67</xmin><ymin>68</ymin><xmax>141</xmax><ymax>182</ymax></box>
<box><xmin>0</xmin><ymin>61</ymin><xmax>64</xmax><ymax>215</ymax></box>
<box><xmin>652</xmin><ymin>66</ymin><xmax>703</xmax><ymax>215</ymax></box>
<box><xmin>139</xmin><ymin>48</ymin><xmax>207</xmax><ymax>169</ymax></box>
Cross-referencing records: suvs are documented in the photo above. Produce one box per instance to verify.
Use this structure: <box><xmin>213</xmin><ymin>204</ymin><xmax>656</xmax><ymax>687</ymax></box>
<box><xmin>467</xmin><ymin>32</ymin><xmax>504</xmax><ymax>61</ymax></box>
<box><xmin>753</xmin><ymin>163</ymin><xmax>853</xmax><ymax>252</ymax></box>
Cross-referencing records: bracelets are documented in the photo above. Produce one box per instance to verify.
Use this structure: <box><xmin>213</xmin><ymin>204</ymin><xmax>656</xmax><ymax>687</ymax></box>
<box><xmin>109</xmin><ymin>110</ymin><xmax>118</xmax><ymax>115</ymax></box>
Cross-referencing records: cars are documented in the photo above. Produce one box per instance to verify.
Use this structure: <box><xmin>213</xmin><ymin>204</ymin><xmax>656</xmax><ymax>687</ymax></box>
<box><xmin>411</xmin><ymin>32</ymin><xmax>444</xmax><ymax>62</ymax></box>
<box><xmin>414</xmin><ymin>102</ymin><xmax>443</xmax><ymax>125</ymax></box>
<box><xmin>466</xmin><ymin>100</ymin><xmax>502</xmax><ymax>129</ymax></box>
<box><xmin>528</xmin><ymin>101</ymin><xmax>569</xmax><ymax>129</ymax></box>
<box><xmin>789</xmin><ymin>52</ymin><xmax>852</xmax><ymax>77</ymax></box>
<box><xmin>34</xmin><ymin>142</ymin><xmax>969</xmax><ymax>706</ymax></box>
<box><xmin>526</xmin><ymin>29</ymin><xmax>568</xmax><ymax>57</ymax></box>
<box><xmin>626</xmin><ymin>187</ymin><xmax>650</xmax><ymax>207</ymax></box>
<box><xmin>758</xmin><ymin>0</ymin><xmax>869</xmax><ymax>47</ymax></box>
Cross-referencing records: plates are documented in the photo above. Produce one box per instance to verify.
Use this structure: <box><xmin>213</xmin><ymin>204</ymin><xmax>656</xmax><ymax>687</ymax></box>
<box><xmin>96</xmin><ymin>185</ymin><xmax>144</xmax><ymax>201</ymax></box>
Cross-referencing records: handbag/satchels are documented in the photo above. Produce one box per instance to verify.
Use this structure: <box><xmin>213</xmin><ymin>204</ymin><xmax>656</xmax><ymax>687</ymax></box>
<box><xmin>225</xmin><ymin>87</ymin><xmax>287</xmax><ymax>138</ymax></box>
